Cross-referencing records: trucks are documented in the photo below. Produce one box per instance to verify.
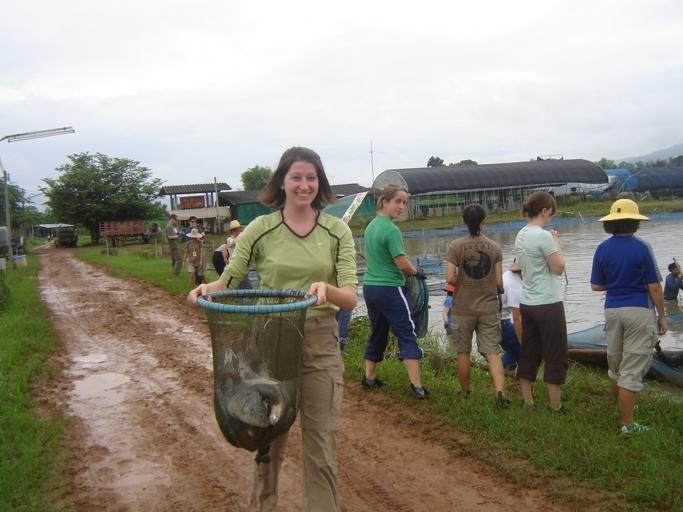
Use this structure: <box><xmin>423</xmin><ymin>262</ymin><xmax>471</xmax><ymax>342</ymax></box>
<box><xmin>98</xmin><ymin>220</ymin><xmax>162</xmax><ymax>247</ymax></box>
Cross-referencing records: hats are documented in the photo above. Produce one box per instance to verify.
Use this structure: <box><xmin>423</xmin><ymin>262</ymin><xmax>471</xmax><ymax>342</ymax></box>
<box><xmin>228</xmin><ymin>220</ymin><xmax>243</xmax><ymax>232</ymax></box>
<box><xmin>186</xmin><ymin>228</ymin><xmax>202</xmax><ymax>238</ymax></box>
<box><xmin>597</xmin><ymin>199</ymin><xmax>651</xmax><ymax>223</ymax></box>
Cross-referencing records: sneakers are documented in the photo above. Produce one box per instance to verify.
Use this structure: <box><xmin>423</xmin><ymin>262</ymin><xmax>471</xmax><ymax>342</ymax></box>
<box><xmin>548</xmin><ymin>405</ymin><xmax>569</xmax><ymax>416</ymax></box>
<box><xmin>411</xmin><ymin>386</ymin><xmax>429</xmax><ymax>399</ymax></box>
<box><xmin>497</xmin><ymin>391</ymin><xmax>509</xmax><ymax>409</ymax></box>
<box><xmin>621</xmin><ymin>424</ymin><xmax>649</xmax><ymax>433</ymax></box>
<box><xmin>362</xmin><ymin>376</ymin><xmax>386</xmax><ymax>388</ymax></box>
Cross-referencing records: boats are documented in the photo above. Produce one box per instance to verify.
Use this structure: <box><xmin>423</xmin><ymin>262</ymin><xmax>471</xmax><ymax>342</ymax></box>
<box><xmin>566</xmin><ymin>311</ymin><xmax>683</xmax><ymax>390</ymax></box>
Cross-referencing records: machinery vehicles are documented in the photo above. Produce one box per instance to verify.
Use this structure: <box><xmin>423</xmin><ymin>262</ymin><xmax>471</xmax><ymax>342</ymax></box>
<box><xmin>55</xmin><ymin>230</ymin><xmax>78</xmax><ymax>248</ymax></box>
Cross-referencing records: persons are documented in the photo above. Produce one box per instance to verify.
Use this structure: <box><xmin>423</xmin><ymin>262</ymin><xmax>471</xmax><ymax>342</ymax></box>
<box><xmin>227</xmin><ymin>220</ymin><xmax>253</xmax><ymax>289</ymax></box>
<box><xmin>442</xmin><ymin>296</ymin><xmax>453</xmax><ymax>335</ymax></box>
<box><xmin>590</xmin><ymin>200</ymin><xmax>668</xmax><ymax>434</ymax></box>
<box><xmin>664</xmin><ymin>263</ymin><xmax>683</xmax><ymax>300</ymax></box>
<box><xmin>166</xmin><ymin>214</ymin><xmax>204</xmax><ymax>283</ymax></box>
<box><xmin>499</xmin><ymin>263</ymin><xmax>523</xmax><ymax>369</ymax></box>
<box><xmin>187</xmin><ymin>146</ymin><xmax>358</xmax><ymax>512</ymax></box>
<box><xmin>336</xmin><ymin>309</ymin><xmax>352</xmax><ymax>356</ymax></box>
<box><xmin>362</xmin><ymin>184</ymin><xmax>429</xmax><ymax>398</ymax></box>
<box><xmin>514</xmin><ymin>191</ymin><xmax>568</xmax><ymax>416</ymax></box>
<box><xmin>444</xmin><ymin>204</ymin><xmax>509</xmax><ymax>408</ymax></box>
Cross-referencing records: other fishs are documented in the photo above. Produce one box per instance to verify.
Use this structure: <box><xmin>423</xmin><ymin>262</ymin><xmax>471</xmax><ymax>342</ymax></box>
<box><xmin>213</xmin><ymin>347</ymin><xmax>297</xmax><ymax>450</ymax></box>
